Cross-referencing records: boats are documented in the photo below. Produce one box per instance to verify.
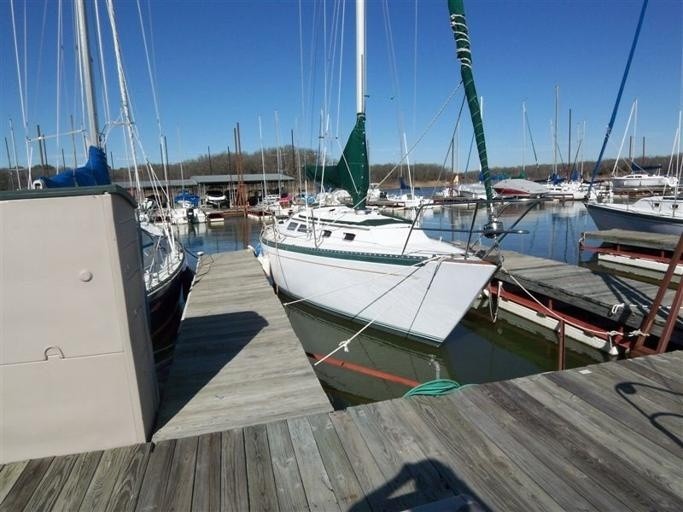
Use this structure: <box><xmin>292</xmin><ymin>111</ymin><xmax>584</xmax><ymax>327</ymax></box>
<box><xmin>169</xmin><ymin>208</ymin><xmax>208</xmax><ymax>224</ymax></box>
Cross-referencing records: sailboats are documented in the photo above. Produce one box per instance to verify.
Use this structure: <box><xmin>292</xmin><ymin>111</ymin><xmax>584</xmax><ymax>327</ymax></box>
<box><xmin>450</xmin><ymin>201</ymin><xmax>586</xmax><ymax>263</ymax></box>
<box><xmin>9</xmin><ymin>0</ymin><xmax>187</xmax><ymax>367</ymax></box>
<box><xmin>258</xmin><ymin>0</ymin><xmax>503</xmax><ymax>346</ymax></box>
<box><xmin>284</xmin><ymin>305</ymin><xmax>459</xmax><ymax>402</ymax></box>
